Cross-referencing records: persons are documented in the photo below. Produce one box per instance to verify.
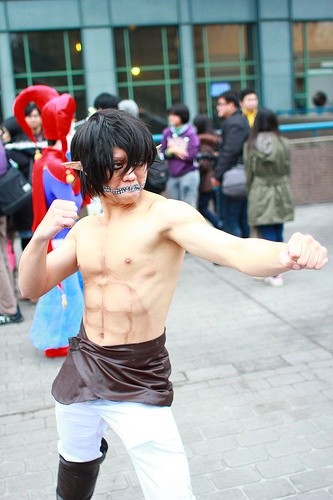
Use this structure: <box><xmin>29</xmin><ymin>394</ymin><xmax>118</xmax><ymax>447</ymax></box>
<box><xmin>0</xmin><ymin>85</ymin><xmax>294</xmax><ymax>358</ymax></box>
<box><xmin>17</xmin><ymin>108</ymin><xmax>328</xmax><ymax>500</ymax></box>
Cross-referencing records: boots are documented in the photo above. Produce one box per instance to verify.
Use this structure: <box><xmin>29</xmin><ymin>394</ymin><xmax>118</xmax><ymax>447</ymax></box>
<box><xmin>56</xmin><ymin>438</ymin><xmax>108</xmax><ymax>500</ymax></box>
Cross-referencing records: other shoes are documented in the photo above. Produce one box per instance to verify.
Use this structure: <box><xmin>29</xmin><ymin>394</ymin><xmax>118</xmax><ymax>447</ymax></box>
<box><xmin>253</xmin><ymin>274</ymin><xmax>284</xmax><ymax>288</ymax></box>
<box><xmin>45</xmin><ymin>346</ymin><xmax>68</xmax><ymax>357</ymax></box>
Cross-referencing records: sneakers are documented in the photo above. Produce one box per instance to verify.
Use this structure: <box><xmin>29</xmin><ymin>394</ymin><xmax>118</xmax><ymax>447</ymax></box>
<box><xmin>0</xmin><ymin>306</ymin><xmax>23</xmax><ymax>326</ymax></box>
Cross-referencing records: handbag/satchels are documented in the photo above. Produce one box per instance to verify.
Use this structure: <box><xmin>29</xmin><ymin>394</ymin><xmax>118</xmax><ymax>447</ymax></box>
<box><xmin>0</xmin><ymin>164</ymin><xmax>32</xmax><ymax>216</ymax></box>
<box><xmin>144</xmin><ymin>160</ymin><xmax>170</xmax><ymax>194</ymax></box>
<box><xmin>222</xmin><ymin>165</ymin><xmax>248</xmax><ymax>196</ymax></box>
